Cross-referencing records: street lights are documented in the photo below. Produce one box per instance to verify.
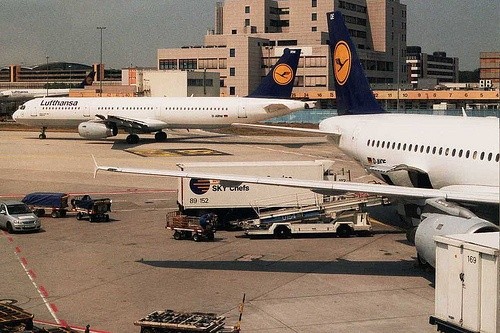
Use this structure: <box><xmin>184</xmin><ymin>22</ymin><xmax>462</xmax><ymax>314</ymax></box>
<box><xmin>96</xmin><ymin>24</ymin><xmax>106</xmax><ymax>97</ymax></box>
<box><xmin>46</xmin><ymin>56</ymin><xmax>50</xmax><ymax>97</ymax></box>
<box><xmin>266</xmin><ymin>46</ymin><xmax>272</xmax><ymax>74</ymax></box>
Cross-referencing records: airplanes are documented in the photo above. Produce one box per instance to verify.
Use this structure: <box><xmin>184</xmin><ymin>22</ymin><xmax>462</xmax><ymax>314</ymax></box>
<box><xmin>0</xmin><ymin>71</ymin><xmax>96</xmax><ymax>108</ymax></box>
<box><xmin>90</xmin><ymin>11</ymin><xmax>500</xmax><ymax>273</ymax></box>
<box><xmin>12</xmin><ymin>48</ymin><xmax>317</xmax><ymax>145</ymax></box>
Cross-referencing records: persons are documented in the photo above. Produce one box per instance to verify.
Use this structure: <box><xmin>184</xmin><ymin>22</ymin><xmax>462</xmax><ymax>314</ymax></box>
<box><xmin>199</xmin><ymin>213</ymin><xmax>213</xmax><ymax>231</ymax></box>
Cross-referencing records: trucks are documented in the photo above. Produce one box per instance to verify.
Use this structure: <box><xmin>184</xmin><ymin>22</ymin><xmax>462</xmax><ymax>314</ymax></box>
<box><xmin>236</xmin><ymin>195</ymin><xmax>384</xmax><ymax>239</ymax></box>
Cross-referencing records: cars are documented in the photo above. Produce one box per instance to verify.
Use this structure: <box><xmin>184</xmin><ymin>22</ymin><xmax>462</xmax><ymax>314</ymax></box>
<box><xmin>0</xmin><ymin>201</ymin><xmax>41</xmax><ymax>234</ymax></box>
